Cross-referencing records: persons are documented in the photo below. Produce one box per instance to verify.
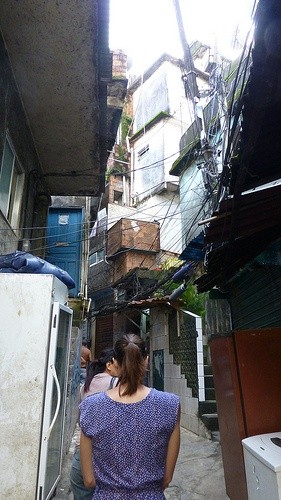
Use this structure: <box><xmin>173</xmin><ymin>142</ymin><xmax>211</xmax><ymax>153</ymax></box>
<box><xmin>77</xmin><ymin>334</ymin><xmax>181</xmax><ymax>500</ymax></box>
<box><xmin>154</xmin><ymin>352</ymin><xmax>165</xmax><ymax>378</ymax></box>
<box><xmin>70</xmin><ymin>348</ymin><xmax>120</xmax><ymax>500</ymax></box>
<box><xmin>80</xmin><ymin>339</ymin><xmax>92</xmax><ymax>386</ymax></box>
